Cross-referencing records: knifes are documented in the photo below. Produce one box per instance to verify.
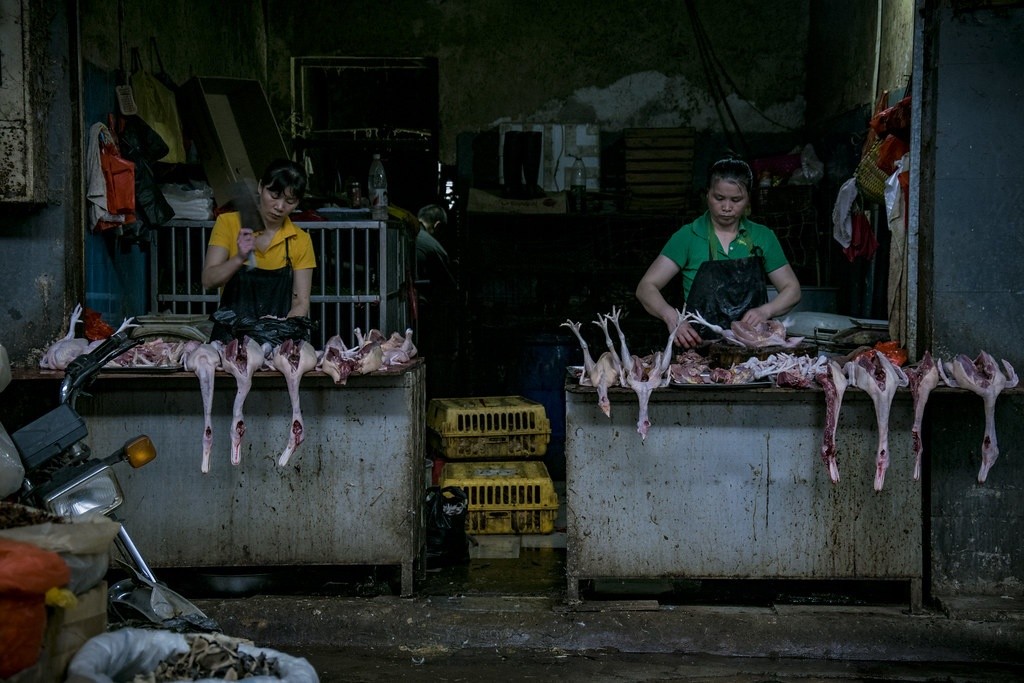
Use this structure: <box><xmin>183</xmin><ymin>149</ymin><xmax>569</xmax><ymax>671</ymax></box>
<box><xmin>239</xmin><ymin>205</ymin><xmax>266</xmax><ymax>269</ymax></box>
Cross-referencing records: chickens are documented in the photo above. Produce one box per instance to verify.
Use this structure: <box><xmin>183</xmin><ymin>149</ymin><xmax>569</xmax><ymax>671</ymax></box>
<box><xmin>40</xmin><ymin>302</ymin><xmax>182</xmax><ymax>368</ymax></box>
<box><xmin>560</xmin><ymin>302</ymin><xmax>820</xmax><ymax>447</ymax></box>
<box><xmin>316</xmin><ymin>326</ymin><xmax>419</xmax><ymax>385</ymax></box>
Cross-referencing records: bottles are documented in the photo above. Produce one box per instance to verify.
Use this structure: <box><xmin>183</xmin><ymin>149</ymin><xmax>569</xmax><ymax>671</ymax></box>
<box><xmin>367</xmin><ymin>154</ymin><xmax>389</xmax><ymax>222</ymax></box>
<box><xmin>349</xmin><ymin>181</ymin><xmax>363</xmax><ymax>209</ymax></box>
<box><xmin>569</xmin><ymin>157</ymin><xmax>588</xmax><ymax>214</ymax></box>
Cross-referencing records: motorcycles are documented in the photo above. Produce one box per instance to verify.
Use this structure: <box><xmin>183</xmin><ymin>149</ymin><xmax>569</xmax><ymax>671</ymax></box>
<box><xmin>0</xmin><ymin>324</ymin><xmax>224</xmax><ymax>636</ymax></box>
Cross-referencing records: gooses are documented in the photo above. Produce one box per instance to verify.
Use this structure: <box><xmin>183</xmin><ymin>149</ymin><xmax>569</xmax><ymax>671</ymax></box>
<box><xmin>820</xmin><ymin>345</ymin><xmax>1019</xmax><ymax>491</ymax></box>
<box><xmin>177</xmin><ymin>335</ymin><xmax>314</xmax><ymax>473</ymax></box>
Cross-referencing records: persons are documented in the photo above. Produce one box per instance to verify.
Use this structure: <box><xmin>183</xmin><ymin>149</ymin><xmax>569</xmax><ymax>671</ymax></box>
<box><xmin>635</xmin><ymin>157</ymin><xmax>803</xmax><ymax>365</ymax></box>
<box><xmin>201</xmin><ymin>158</ymin><xmax>317</xmax><ymax>346</ymax></box>
<box><xmin>410</xmin><ymin>204</ymin><xmax>449</xmax><ymax>276</ymax></box>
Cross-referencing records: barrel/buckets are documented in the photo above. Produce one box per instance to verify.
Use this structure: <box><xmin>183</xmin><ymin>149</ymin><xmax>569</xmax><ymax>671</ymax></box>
<box><xmin>426</xmin><ymin>459</ymin><xmax>433</xmax><ymax>488</ymax></box>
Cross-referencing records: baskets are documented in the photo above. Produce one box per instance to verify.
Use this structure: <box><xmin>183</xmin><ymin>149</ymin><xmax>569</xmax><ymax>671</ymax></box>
<box><xmin>855</xmin><ymin>139</ymin><xmax>893</xmax><ymax>205</ymax></box>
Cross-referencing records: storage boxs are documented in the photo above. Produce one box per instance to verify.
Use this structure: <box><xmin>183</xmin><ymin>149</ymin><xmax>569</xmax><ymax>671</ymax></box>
<box><xmin>440</xmin><ymin>463</ymin><xmax>560</xmax><ymax>535</ymax></box>
<box><xmin>426</xmin><ymin>395</ymin><xmax>552</xmax><ymax>460</ymax></box>
<box><xmin>42</xmin><ymin>579</ymin><xmax>109</xmax><ymax>683</ymax></box>
<box><xmin>177</xmin><ymin>77</ymin><xmax>290</xmax><ymax>208</ymax></box>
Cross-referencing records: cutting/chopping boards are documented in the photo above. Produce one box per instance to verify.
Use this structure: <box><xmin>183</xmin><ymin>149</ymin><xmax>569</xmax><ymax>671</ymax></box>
<box><xmin>710</xmin><ymin>341</ymin><xmax>819</xmax><ymax>369</ymax></box>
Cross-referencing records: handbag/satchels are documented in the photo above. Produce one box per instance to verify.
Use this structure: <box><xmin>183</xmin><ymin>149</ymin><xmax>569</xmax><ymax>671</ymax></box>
<box><xmin>210</xmin><ymin>304</ymin><xmax>311</xmax><ymax>349</ymax></box>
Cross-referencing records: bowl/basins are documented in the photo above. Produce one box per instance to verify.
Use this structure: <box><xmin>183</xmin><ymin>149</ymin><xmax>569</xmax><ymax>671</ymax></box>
<box><xmin>202</xmin><ymin>573</ymin><xmax>265</xmax><ymax>596</ymax></box>
